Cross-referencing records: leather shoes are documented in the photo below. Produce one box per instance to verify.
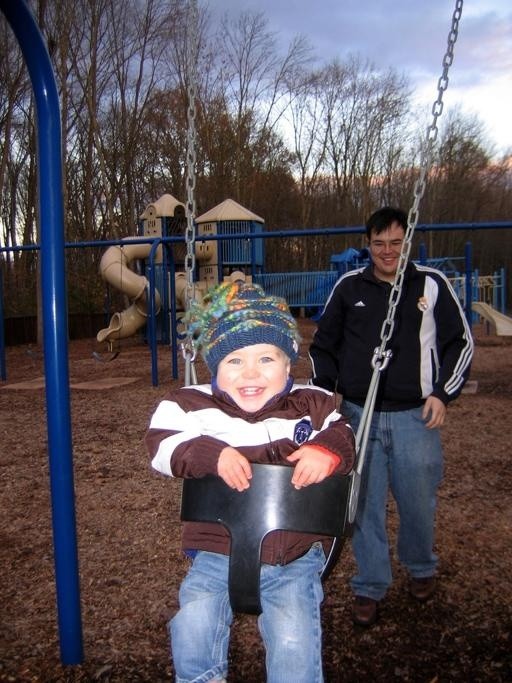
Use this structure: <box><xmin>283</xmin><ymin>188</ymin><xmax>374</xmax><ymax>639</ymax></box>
<box><xmin>352</xmin><ymin>596</ymin><xmax>379</xmax><ymax>626</ymax></box>
<box><xmin>408</xmin><ymin>576</ymin><xmax>436</xmax><ymax>599</ymax></box>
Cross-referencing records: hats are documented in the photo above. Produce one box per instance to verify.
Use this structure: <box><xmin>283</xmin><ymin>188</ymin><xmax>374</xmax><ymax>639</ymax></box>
<box><xmin>185</xmin><ymin>280</ymin><xmax>303</xmax><ymax>374</ymax></box>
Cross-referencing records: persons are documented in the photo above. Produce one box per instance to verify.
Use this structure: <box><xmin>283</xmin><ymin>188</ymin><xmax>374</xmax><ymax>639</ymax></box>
<box><xmin>146</xmin><ymin>285</ymin><xmax>357</xmax><ymax>683</ymax></box>
<box><xmin>307</xmin><ymin>207</ymin><xmax>475</xmax><ymax>626</ymax></box>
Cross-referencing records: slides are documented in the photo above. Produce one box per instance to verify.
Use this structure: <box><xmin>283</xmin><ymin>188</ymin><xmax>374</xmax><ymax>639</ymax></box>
<box><xmin>472</xmin><ymin>301</ymin><xmax>512</xmax><ymax>337</ymax></box>
<box><xmin>96</xmin><ymin>237</ymin><xmax>160</xmax><ymax>342</ymax></box>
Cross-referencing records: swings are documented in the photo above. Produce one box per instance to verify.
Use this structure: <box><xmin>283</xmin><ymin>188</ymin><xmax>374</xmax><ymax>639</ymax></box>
<box><xmin>180</xmin><ymin>0</ymin><xmax>462</xmax><ymax>616</ymax></box>
